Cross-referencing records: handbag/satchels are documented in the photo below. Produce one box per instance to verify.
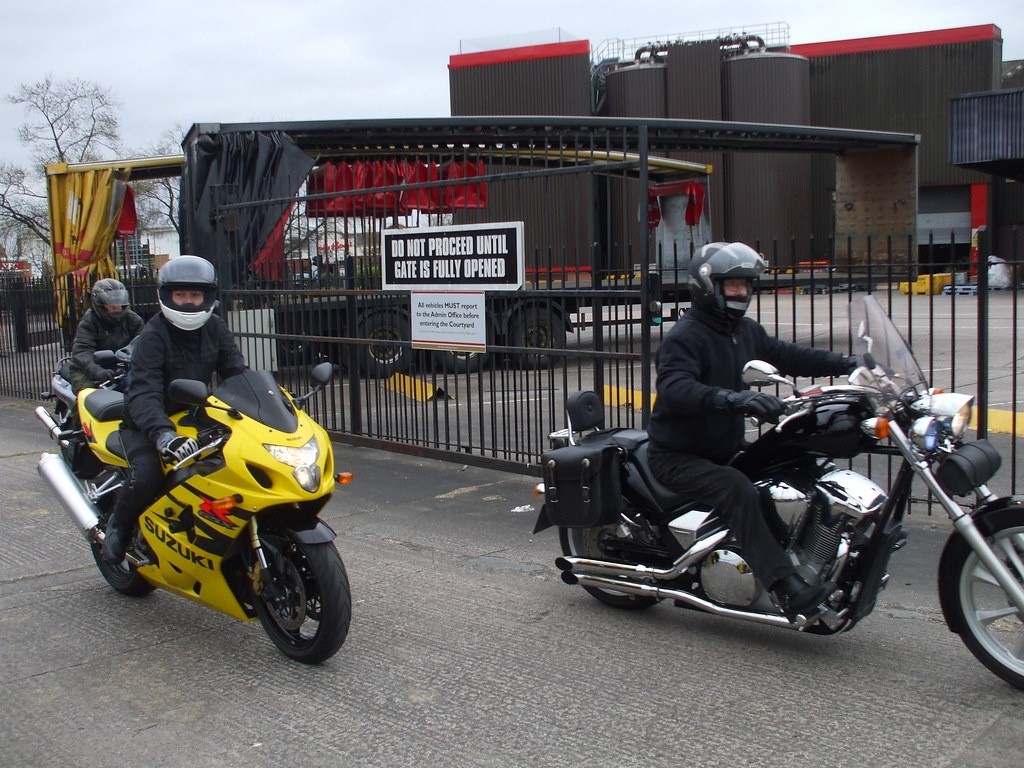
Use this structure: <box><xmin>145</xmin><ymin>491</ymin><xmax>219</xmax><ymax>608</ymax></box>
<box><xmin>540</xmin><ymin>443</ymin><xmax>622</xmax><ymax>529</ymax></box>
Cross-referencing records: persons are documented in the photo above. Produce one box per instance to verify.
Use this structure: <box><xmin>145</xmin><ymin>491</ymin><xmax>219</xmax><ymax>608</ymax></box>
<box><xmin>71</xmin><ymin>277</ymin><xmax>145</xmax><ymax>447</ymax></box>
<box><xmin>95</xmin><ymin>253</ymin><xmax>249</xmax><ymax>565</ymax></box>
<box><xmin>647</xmin><ymin>242</ymin><xmax>896</xmax><ymax>625</ymax></box>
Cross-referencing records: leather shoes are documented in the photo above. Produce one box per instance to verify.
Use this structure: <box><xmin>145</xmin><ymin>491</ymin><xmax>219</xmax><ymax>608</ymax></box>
<box><xmin>103</xmin><ymin>513</ymin><xmax>132</xmax><ymax>568</ymax></box>
<box><xmin>772</xmin><ymin>571</ymin><xmax>838</xmax><ymax>624</ymax></box>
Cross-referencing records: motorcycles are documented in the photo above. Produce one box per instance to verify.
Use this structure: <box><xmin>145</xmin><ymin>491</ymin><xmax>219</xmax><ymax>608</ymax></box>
<box><xmin>38</xmin><ymin>361</ymin><xmax>353</xmax><ymax>664</ymax></box>
<box><xmin>36</xmin><ymin>335</ymin><xmax>131</xmax><ymax>477</ymax></box>
<box><xmin>533</xmin><ymin>295</ymin><xmax>1024</xmax><ymax>689</ymax></box>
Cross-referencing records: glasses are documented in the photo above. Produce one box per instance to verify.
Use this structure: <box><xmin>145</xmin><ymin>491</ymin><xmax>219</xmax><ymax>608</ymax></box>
<box><xmin>725</xmin><ymin>281</ymin><xmax>748</xmax><ymax>291</ymax></box>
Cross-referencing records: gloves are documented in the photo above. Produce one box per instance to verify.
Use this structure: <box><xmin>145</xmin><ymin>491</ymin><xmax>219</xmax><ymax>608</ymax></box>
<box><xmin>712</xmin><ymin>388</ymin><xmax>786</xmax><ymax>425</ymax></box>
<box><xmin>90</xmin><ymin>363</ymin><xmax>116</xmax><ymax>386</ymax></box>
<box><xmin>838</xmin><ymin>354</ymin><xmax>895</xmax><ymax>384</ymax></box>
<box><xmin>152</xmin><ymin>428</ymin><xmax>198</xmax><ymax>464</ymax></box>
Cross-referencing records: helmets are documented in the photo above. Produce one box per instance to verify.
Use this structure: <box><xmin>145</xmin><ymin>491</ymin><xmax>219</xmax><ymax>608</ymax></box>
<box><xmin>687</xmin><ymin>242</ymin><xmax>767</xmax><ymax>333</ymax></box>
<box><xmin>156</xmin><ymin>255</ymin><xmax>218</xmax><ymax>331</ymax></box>
<box><xmin>90</xmin><ymin>278</ymin><xmax>130</xmax><ymax>328</ymax></box>
